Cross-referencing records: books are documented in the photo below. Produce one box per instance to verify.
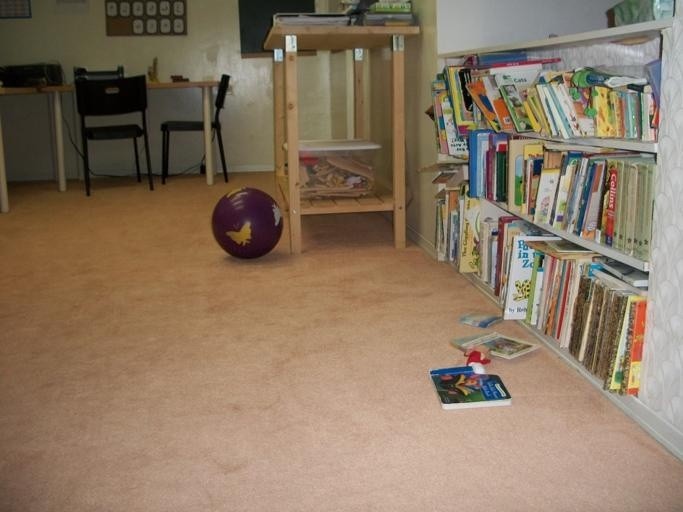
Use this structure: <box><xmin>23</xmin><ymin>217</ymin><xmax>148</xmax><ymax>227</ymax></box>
<box><xmin>423</xmin><ymin>49</ymin><xmax>661</xmax><ymax>161</ymax></box>
<box><xmin>429</xmin><ymin>373</ymin><xmax>513</xmax><ymax>413</ymax></box>
<box><xmin>436</xmin><ymin>193</ymin><xmax>648</xmax><ymax>397</ymax></box>
<box><xmin>270</xmin><ymin>0</ymin><xmax>417</xmax><ymax>27</ymax></box>
<box><xmin>431</xmin><ymin>133</ymin><xmax>656</xmax><ymax>261</ymax></box>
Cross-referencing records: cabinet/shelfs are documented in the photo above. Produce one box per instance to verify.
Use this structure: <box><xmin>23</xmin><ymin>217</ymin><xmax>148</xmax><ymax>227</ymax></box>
<box><xmin>433</xmin><ymin>16</ymin><xmax>674</xmax><ymax>456</ymax></box>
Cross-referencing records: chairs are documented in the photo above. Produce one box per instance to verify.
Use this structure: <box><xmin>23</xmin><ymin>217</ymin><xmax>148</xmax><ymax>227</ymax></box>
<box><xmin>160</xmin><ymin>73</ymin><xmax>233</xmax><ymax>188</ymax></box>
<box><xmin>74</xmin><ymin>74</ymin><xmax>155</xmax><ymax>197</ymax></box>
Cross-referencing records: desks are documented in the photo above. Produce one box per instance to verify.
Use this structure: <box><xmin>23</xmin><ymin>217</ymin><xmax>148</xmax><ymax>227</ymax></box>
<box><xmin>0</xmin><ymin>79</ymin><xmax>225</xmax><ymax>190</ymax></box>
<box><xmin>263</xmin><ymin>21</ymin><xmax>423</xmax><ymax>257</ymax></box>
